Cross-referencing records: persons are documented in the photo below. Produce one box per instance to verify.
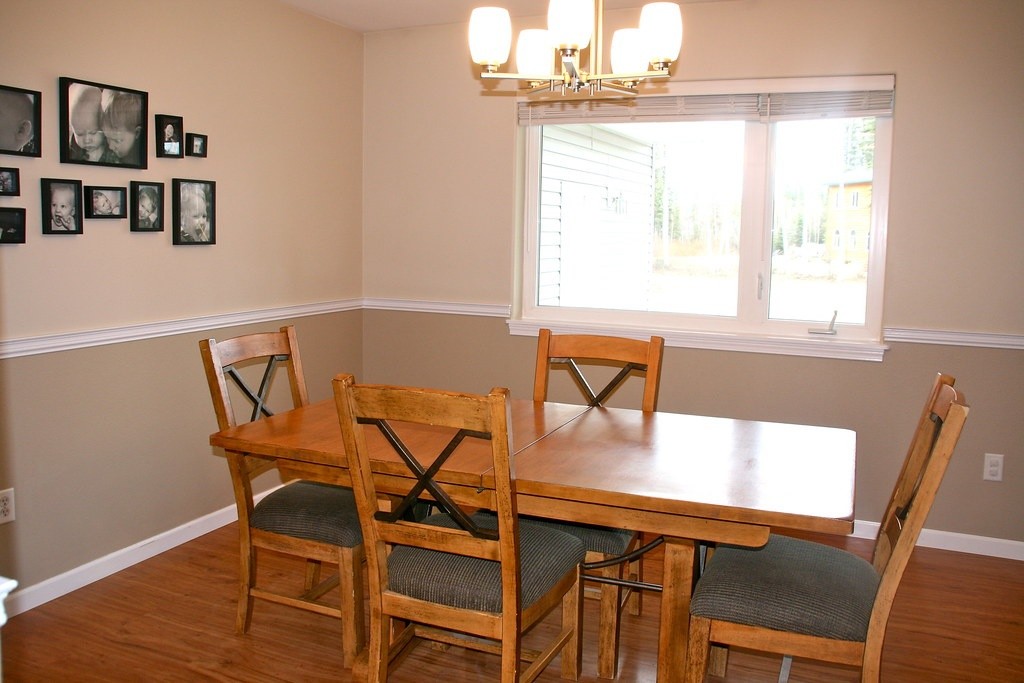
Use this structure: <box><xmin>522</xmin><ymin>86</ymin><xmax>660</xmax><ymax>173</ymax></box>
<box><xmin>0</xmin><ymin>90</ymin><xmax>35</xmax><ymax>152</ymax></box>
<box><xmin>181</xmin><ymin>184</ymin><xmax>209</xmax><ymax>241</ymax></box>
<box><xmin>68</xmin><ymin>83</ymin><xmax>142</xmax><ymax>165</ymax></box>
<box><xmin>93</xmin><ymin>192</ymin><xmax>120</xmax><ymax>215</ymax></box>
<box><xmin>51</xmin><ymin>185</ymin><xmax>77</xmax><ymax>230</ymax></box>
<box><xmin>164</xmin><ymin>124</ymin><xmax>175</xmax><ymax>142</ymax></box>
<box><xmin>139</xmin><ymin>187</ymin><xmax>159</xmax><ymax>228</ymax></box>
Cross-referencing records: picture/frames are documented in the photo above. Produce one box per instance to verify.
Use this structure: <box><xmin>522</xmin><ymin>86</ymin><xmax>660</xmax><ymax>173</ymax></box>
<box><xmin>0</xmin><ymin>77</ymin><xmax>216</xmax><ymax>246</ymax></box>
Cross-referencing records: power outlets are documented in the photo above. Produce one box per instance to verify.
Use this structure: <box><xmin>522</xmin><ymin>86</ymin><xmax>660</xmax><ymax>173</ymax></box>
<box><xmin>0</xmin><ymin>487</ymin><xmax>16</xmax><ymax>525</ymax></box>
<box><xmin>983</xmin><ymin>452</ymin><xmax>1004</xmax><ymax>482</ymax></box>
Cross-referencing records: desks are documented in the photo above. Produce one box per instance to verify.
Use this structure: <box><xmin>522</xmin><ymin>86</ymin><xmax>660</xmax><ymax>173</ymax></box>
<box><xmin>211</xmin><ymin>398</ymin><xmax>857</xmax><ymax>683</ymax></box>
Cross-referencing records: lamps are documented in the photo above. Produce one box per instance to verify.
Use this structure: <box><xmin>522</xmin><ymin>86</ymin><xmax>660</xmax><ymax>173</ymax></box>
<box><xmin>467</xmin><ymin>0</ymin><xmax>683</xmax><ymax>103</ymax></box>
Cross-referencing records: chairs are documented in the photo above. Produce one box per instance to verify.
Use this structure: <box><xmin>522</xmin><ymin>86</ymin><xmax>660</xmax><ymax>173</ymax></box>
<box><xmin>199</xmin><ymin>324</ymin><xmax>970</xmax><ymax>683</ymax></box>
